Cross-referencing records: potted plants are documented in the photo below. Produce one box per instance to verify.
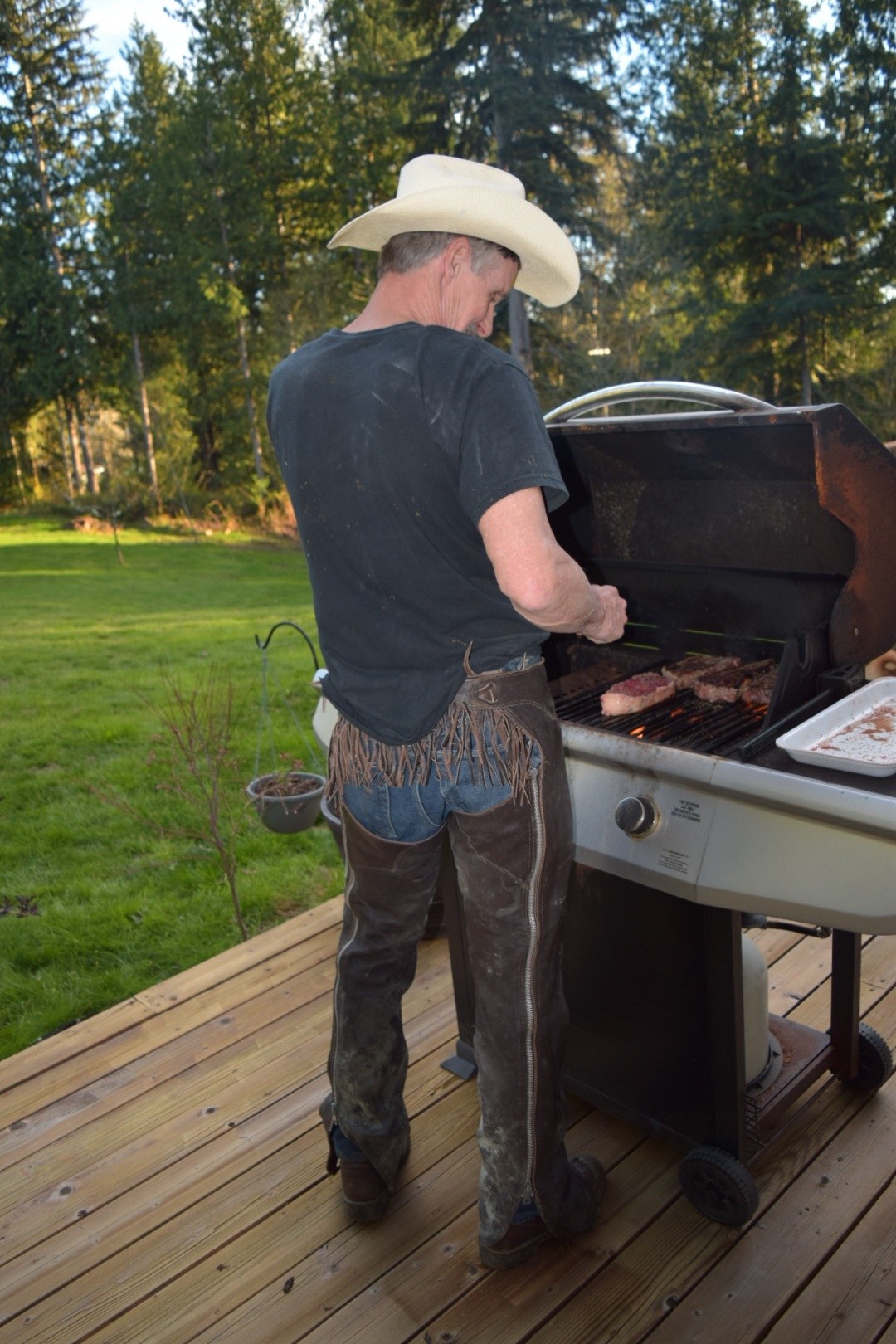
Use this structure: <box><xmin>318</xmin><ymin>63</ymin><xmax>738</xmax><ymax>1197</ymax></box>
<box><xmin>248</xmin><ymin>750</ymin><xmax>328</xmax><ymax>835</ymax></box>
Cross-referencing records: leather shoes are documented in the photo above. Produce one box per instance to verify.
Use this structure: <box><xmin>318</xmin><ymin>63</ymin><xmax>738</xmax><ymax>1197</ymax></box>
<box><xmin>480</xmin><ymin>1157</ymin><xmax>608</xmax><ymax>1271</ymax></box>
<box><xmin>341</xmin><ymin>1158</ymin><xmax>391</xmax><ymax>1224</ymax></box>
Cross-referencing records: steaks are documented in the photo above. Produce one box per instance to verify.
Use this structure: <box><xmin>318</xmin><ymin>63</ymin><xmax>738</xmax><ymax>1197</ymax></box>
<box><xmin>598</xmin><ymin>652</ymin><xmax>782</xmax><ymax>715</ymax></box>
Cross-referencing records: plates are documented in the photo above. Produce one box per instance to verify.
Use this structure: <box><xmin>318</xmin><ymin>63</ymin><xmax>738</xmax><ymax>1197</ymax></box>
<box><xmin>776</xmin><ymin>676</ymin><xmax>896</xmax><ymax>777</ymax></box>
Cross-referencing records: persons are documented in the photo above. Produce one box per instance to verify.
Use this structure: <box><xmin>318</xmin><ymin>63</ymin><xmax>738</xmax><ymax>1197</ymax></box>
<box><xmin>267</xmin><ymin>154</ymin><xmax>628</xmax><ymax>1272</ymax></box>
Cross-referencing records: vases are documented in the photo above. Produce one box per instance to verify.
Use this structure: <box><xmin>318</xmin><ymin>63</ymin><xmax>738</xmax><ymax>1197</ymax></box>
<box><xmin>319</xmin><ymin>782</ymin><xmax>347</xmax><ymax>862</ymax></box>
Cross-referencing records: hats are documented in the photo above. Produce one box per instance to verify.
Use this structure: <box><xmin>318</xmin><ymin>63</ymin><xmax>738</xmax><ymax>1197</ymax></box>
<box><xmin>328</xmin><ymin>151</ymin><xmax>582</xmax><ymax>308</ymax></box>
